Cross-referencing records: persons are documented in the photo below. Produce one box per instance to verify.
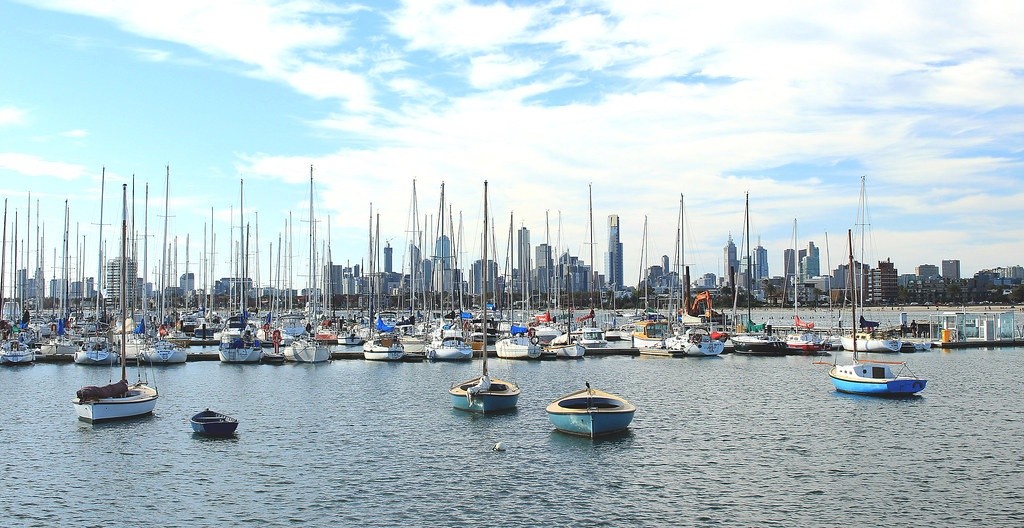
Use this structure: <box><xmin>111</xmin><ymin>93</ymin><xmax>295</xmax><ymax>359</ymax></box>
<box><xmin>910</xmin><ymin>319</ymin><xmax>917</xmax><ymax>338</ymax></box>
<box><xmin>901</xmin><ymin>320</ymin><xmax>908</xmax><ymax>338</ymax></box>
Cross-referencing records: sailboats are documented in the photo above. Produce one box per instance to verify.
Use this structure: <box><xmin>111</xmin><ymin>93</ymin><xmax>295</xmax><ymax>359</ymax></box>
<box><xmin>812</xmin><ymin>227</ymin><xmax>930</xmax><ymax>395</ymax></box>
<box><xmin>72</xmin><ymin>184</ymin><xmax>160</xmax><ymax>424</ymax></box>
<box><xmin>0</xmin><ymin>160</ymin><xmax>930</xmax><ymax>368</ymax></box>
<box><xmin>449</xmin><ymin>179</ymin><xmax>521</xmax><ymax>412</ymax></box>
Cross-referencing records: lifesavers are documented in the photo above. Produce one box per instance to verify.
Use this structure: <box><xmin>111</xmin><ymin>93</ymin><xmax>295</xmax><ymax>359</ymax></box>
<box><xmin>264</xmin><ymin>324</ymin><xmax>269</xmax><ymax>329</ymax></box>
<box><xmin>11</xmin><ymin>342</ymin><xmax>19</xmax><ymax>351</ymax></box>
<box><xmin>529</xmin><ymin>328</ymin><xmax>535</xmax><ymax>336</ymax></box>
<box><xmin>531</xmin><ymin>336</ymin><xmax>539</xmax><ymax>344</ymax></box>
<box><xmin>273</xmin><ymin>330</ymin><xmax>282</xmax><ymax>343</ymax></box>
<box><xmin>51</xmin><ymin>324</ymin><xmax>56</xmax><ymax>331</ymax></box>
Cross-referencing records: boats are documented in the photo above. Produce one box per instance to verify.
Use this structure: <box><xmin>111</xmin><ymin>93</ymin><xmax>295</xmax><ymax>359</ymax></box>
<box><xmin>545</xmin><ymin>380</ymin><xmax>637</xmax><ymax>438</ymax></box>
<box><xmin>189</xmin><ymin>408</ymin><xmax>240</xmax><ymax>436</ymax></box>
